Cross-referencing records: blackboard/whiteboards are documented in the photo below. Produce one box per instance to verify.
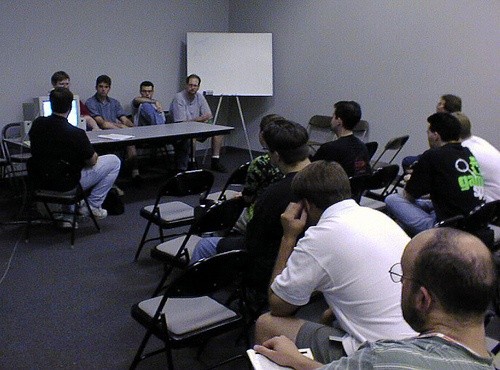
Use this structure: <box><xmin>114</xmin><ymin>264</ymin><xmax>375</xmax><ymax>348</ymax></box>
<box><xmin>186</xmin><ymin>31</ymin><xmax>273</xmax><ymax>97</ymax></box>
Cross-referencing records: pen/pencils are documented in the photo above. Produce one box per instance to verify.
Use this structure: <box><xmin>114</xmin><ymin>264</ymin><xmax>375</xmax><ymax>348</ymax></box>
<box><xmin>256</xmin><ymin>351</ymin><xmax>308</xmax><ymax>354</ymax></box>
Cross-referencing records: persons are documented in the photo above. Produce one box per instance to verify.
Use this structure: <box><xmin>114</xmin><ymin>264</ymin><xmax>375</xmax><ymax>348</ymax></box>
<box><xmin>50</xmin><ymin>71</ymin><xmax>226</xmax><ymax>188</ymax></box>
<box><xmin>256</xmin><ymin>160</ymin><xmax>421</xmax><ymax>364</ymax></box>
<box><xmin>253</xmin><ymin>228</ymin><xmax>497</xmax><ymax>370</ymax></box>
<box><xmin>188</xmin><ymin>114</ymin><xmax>312</xmax><ymax>265</ymax></box>
<box><xmin>308</xmin><ymin>100</ymin><xmax>370</xmax><ymax>177</ymax></box>
<box><xmin>28</xmin><ymin>86</ymin><xmax>121</xmax><ymax>229</ymax></box>
<box><xmin>385</xmin><ymin>94</ymin><xmax>500</xmax><ymax>233</ymax></box>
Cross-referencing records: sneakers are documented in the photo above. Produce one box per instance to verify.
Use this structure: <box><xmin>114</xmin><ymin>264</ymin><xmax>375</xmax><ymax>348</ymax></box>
<box><xmin>61</xmin><ymin>216</ymin><xmax>79</xmax><ymax>230</ymax></box>
<box><xmin>77</xmin><ymin>202</ymin><xmax>108</xmax><ymax>220</ymax></box>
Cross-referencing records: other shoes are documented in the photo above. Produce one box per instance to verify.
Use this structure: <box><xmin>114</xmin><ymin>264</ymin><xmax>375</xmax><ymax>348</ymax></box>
<box><xmin>120</xmin><ymin>173</ymin><xmax>131</xmax><ymax>188</ymax></box>
<box><xmin>186</xmin><ymin>159</ymin><xmax>197</xmax><ymax>173</ymax></box>
<box><xmin>210</xmin><ymin>157</ymin><xmax>229</xmax><ymax>174</ymax></box>
<box><xmin>110</xmin><ymin>183</ymin><xmax>124</xmax><ymax>196</ymax></box>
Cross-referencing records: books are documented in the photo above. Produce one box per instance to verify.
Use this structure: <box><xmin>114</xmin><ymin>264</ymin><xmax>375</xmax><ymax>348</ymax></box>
<box><xmin>246</xmin><ymin>348</ymin><xmax>315</xmax><ymax>370</ymax></box>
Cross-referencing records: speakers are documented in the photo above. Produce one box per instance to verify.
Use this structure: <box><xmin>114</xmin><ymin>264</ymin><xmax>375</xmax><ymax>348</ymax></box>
<box><xmin>79</xmin><ymin>117</ymin><xmax>86</xmax><ymax>131</ymax></box>
<box><xmin>24</xmin><ymin>120</ymin><xmax>32</xmax><ymax>134</ymax></box>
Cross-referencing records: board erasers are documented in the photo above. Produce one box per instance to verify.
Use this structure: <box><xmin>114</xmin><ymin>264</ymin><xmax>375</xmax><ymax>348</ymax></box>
<box><xmin>203</xmin><ymin>90</ymin><xmax>213</xmax><ymax>96</ymax></box>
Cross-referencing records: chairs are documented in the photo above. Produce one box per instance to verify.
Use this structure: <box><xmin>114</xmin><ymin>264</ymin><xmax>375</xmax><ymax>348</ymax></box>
<box><xmin>127</xmin><ymin>161</ymin><xmax>264</xmax><ymax>370</ymax></box>
<box><xmin>303</xmin><ymin>110</ymin><xmax>500</xmax><ymax>234</ymax></box>
<box><xmin>0</xmin><ymin>122</ymin><xmax>35</xmax><ymax>202</ymax></box>
<box><xmin>24</xmin><ymin>155</ymin><xmax>103</xmax><ymax>243</ymax></box>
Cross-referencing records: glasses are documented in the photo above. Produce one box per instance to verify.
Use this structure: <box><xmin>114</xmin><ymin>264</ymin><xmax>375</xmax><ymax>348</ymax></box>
<box><xmin>141</xmin><ymin>88</ymin><xmax>153</xmax><ymax>95</ymax></box>
<box><xmin>388</xmin><ymin>262</ymin><xmax>432</xmax><ymax>296</ymax></box>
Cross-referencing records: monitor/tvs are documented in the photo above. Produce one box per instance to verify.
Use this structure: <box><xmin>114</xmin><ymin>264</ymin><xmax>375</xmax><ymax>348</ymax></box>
<box><xmin>37</xmin><ymin>94</ymin><xmax>81</xmax><ymax>129</ymax></box>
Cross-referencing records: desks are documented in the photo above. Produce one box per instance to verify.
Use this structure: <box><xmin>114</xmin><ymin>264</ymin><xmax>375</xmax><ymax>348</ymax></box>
<box><xmin>2</xmin><ymin>120</ymin><xmax>235</xmax><ymax>169</ymax></box>
